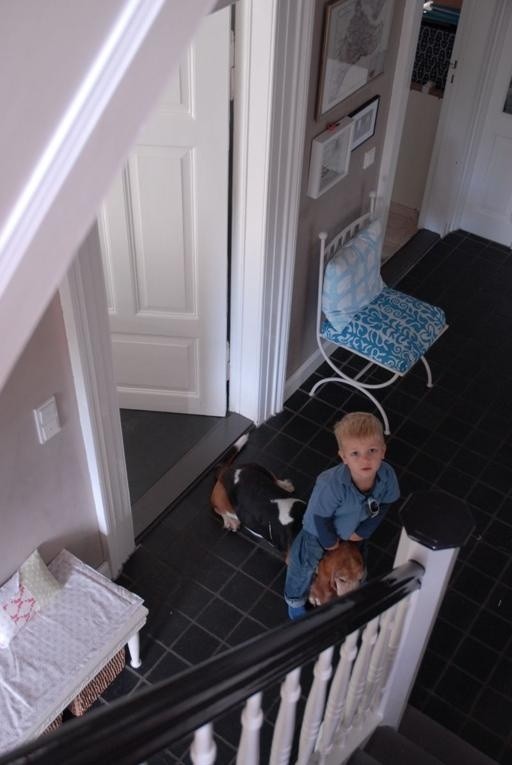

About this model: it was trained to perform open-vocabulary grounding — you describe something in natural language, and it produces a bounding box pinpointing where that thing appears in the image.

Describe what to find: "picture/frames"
[307,0,383,202]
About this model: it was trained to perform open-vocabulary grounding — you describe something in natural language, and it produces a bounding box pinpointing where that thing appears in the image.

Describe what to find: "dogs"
[208,431,369,609]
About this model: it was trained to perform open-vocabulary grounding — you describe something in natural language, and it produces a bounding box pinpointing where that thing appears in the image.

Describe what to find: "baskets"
[68,649,126,717]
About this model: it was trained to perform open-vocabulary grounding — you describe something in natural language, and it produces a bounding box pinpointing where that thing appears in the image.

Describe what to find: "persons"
[283,409,404,618]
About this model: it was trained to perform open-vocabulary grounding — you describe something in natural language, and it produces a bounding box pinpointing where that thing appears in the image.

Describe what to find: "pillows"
[1,550,63,645]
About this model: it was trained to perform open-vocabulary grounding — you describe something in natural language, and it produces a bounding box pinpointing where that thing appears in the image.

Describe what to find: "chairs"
[309,188,445,436]
